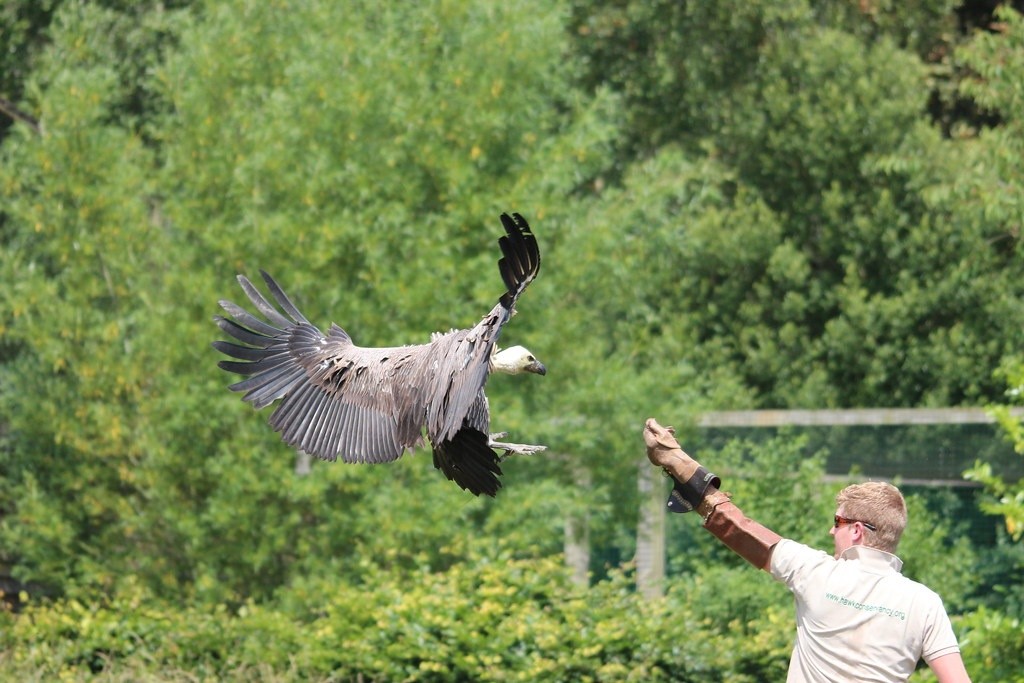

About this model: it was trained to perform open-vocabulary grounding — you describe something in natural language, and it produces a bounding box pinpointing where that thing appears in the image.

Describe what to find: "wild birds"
[215,209,546,497]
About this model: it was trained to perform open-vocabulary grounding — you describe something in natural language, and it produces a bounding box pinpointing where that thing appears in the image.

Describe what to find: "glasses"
[834,514,878,531]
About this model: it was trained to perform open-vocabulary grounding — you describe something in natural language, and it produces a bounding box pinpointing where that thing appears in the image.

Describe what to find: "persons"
[644,418,973,683]
